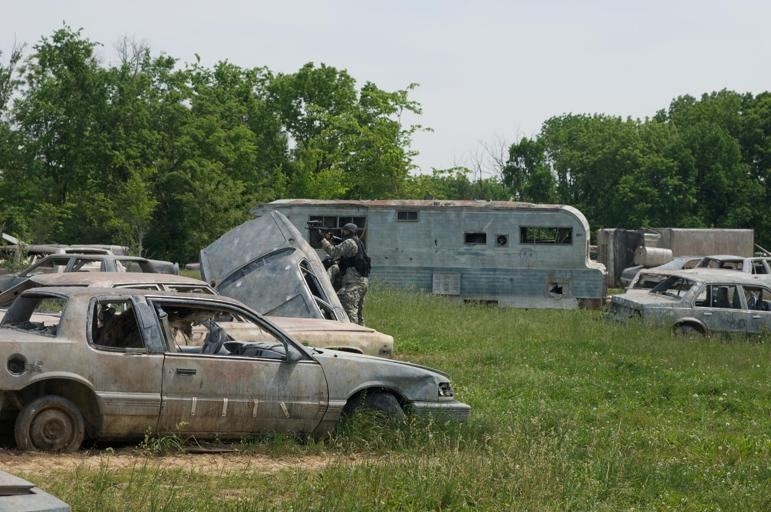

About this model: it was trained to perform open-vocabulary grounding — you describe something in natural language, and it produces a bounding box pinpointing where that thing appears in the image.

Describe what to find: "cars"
[604,254,771,342]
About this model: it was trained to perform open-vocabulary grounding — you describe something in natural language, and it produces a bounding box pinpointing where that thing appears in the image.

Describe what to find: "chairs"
[733,288,756,310]
[752,265,767,273]
[716,286,731,308]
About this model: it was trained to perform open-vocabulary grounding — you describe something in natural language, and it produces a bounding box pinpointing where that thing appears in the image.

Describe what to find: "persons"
[288,221,372,327]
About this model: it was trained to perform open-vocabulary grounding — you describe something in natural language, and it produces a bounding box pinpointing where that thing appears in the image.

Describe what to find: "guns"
[304,220,342,231]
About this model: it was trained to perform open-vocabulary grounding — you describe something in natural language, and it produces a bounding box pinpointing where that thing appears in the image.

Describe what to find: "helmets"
[342,223,359,233]
